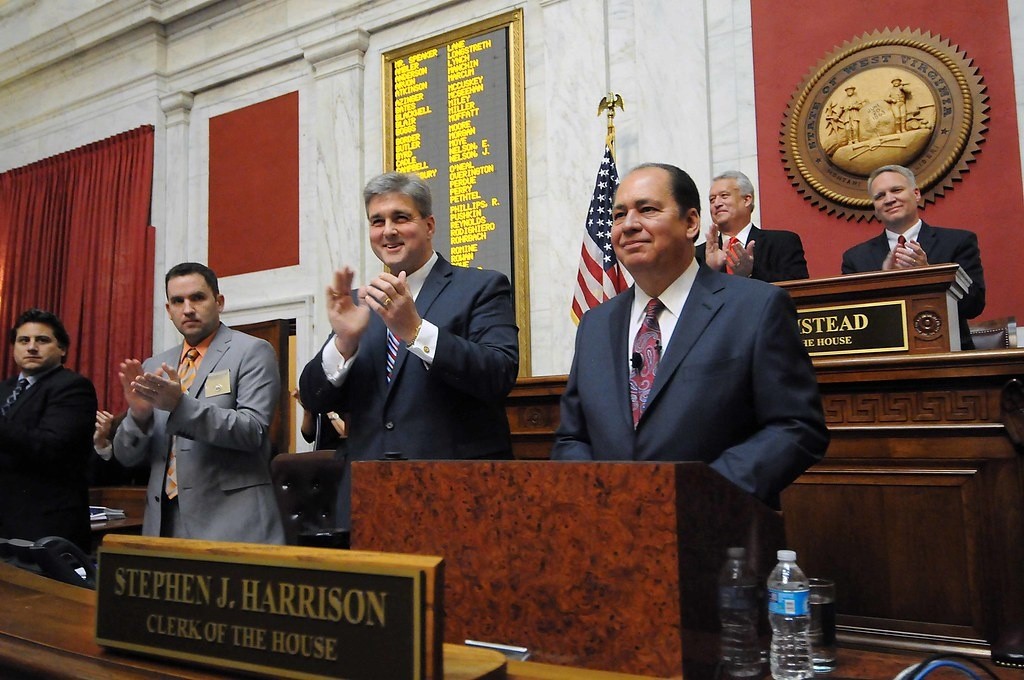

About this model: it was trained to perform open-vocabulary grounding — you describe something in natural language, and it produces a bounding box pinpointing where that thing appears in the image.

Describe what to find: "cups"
[809,578,836,673]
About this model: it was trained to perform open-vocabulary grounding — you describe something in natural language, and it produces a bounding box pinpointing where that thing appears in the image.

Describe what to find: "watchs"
[329,413,339,421]
[407,324,421,348]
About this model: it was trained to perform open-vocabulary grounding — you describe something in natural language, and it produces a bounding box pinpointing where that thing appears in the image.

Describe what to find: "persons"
[292,388,350,453]
[550,162,829,510]
[695,170,810,283]
[0,308,98,552]
[841,164,987,351]
[113,263,284,546]
[87,410,151,487]
[298,172,520,515]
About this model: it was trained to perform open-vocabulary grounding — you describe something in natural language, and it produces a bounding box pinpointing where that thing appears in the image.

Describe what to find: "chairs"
[271,450,340,544]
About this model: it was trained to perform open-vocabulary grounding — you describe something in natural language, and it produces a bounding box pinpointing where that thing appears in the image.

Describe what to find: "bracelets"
[748,274,752,278]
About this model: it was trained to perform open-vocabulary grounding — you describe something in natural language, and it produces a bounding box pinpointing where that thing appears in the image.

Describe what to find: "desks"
[0,559,660,680]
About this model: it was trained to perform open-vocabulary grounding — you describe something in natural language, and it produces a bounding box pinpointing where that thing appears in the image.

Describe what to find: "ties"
[385,331,400,382]
[0,378,29,417]
[165,349,200,500]
[726,237,738,275]
[630,298,666,429]
[898,236,906,250]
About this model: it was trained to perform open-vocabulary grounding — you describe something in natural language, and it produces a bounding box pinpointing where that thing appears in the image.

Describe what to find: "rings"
[736,263,740,266]
[919,249,922,255]
[383,298,390,305]
[333,291,340,296]
[912,260,916,264]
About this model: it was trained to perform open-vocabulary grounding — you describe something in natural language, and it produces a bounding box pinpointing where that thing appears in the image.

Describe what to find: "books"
[89,506,126,521]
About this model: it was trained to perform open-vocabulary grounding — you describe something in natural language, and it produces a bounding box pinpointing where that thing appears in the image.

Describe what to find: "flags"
[570,139,628,326]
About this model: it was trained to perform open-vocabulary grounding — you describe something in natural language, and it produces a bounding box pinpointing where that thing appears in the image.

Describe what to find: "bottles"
[764,550,813,680]
[720,547,761,676]
